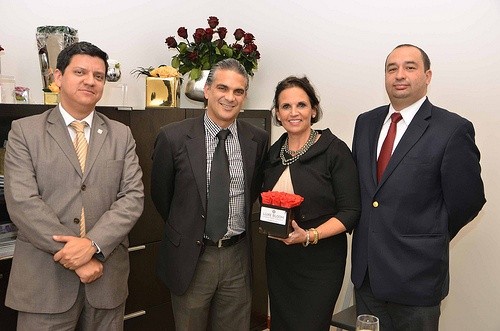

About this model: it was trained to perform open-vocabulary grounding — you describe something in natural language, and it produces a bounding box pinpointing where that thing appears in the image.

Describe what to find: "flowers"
[134,62,181,110]
[261,190,305,208]
[166,18,261,110]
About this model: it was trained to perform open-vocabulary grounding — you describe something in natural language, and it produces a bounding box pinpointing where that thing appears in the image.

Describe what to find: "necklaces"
[280,128,318,166]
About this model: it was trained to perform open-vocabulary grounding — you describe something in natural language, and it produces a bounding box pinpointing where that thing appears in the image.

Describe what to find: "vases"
[146,76,180,110]
[259,204,299,239]
[183,70,224,109]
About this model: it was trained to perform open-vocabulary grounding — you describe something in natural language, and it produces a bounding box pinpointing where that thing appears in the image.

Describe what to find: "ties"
[68,120,88,237]
[377,112,403,185]
[204,130,229,243]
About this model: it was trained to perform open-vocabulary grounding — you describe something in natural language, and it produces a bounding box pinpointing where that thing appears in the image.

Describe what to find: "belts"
[204,232,246,247]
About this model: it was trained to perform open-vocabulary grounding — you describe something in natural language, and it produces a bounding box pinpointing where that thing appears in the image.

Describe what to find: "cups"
[356,314,379,331]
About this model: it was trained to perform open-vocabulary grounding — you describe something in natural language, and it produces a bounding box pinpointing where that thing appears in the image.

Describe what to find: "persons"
[352,44,487,331]
[258,75,362,331]
[149,58,272,331]
[3,42,144,331]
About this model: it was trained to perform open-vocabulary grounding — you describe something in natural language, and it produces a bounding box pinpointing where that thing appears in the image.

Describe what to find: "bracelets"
[309,228,319,244]
[91,241,101,253]
[303,229,310,247]
